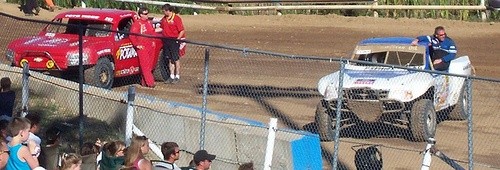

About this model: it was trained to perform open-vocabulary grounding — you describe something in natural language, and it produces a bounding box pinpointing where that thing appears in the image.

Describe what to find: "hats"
[194,150,216,163]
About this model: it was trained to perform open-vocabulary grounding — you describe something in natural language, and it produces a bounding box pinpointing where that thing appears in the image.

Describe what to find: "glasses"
[437,33,446,36]
[204,159,211,162]
[142,13,148,15]
[2,150,11,156]
[175,151,179,154]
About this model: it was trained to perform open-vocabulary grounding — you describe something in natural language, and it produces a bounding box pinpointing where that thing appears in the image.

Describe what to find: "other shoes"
[36,7,41,13]
[175,75,180,80]
[170,74,175,80]
[51,6,55,11]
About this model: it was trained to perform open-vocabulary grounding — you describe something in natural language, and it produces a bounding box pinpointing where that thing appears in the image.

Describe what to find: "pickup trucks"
[4,8,170,90]
[314,37,475,141]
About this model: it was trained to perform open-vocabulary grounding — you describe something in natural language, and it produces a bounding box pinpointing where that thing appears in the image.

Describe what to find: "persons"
[57,152,83,170]
[80,143,98,170]
[0,136,11,170]
[0,76,17,121]
[22,112,42,158]
[129,7,158,89]
[410,26,457,72]
[4,115,39,170]
[37,126,66,170]
[160,141,182,170]
[192,150,212,170]
[93,137,128,170]
[155,3,185,84]
[0,119,10,143]
[123,135,154,170]
[24,0,41,16]
